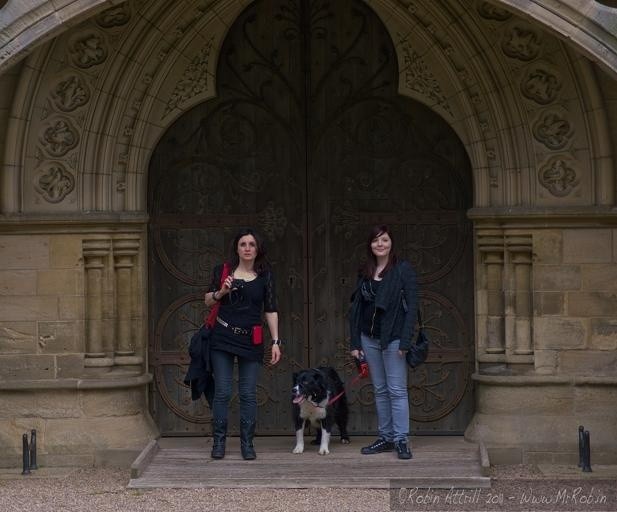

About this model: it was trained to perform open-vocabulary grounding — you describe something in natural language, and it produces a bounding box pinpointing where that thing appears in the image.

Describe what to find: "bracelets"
[271,339,281,345]
[212,291,220,301]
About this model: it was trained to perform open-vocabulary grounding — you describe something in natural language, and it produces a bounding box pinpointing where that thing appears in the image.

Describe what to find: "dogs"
[291,367,350,455]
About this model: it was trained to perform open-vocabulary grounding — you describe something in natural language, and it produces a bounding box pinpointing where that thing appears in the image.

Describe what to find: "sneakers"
[396,440,412,459]
[361,440,395,454]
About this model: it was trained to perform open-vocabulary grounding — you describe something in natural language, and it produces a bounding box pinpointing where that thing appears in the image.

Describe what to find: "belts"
[217,316,250,336]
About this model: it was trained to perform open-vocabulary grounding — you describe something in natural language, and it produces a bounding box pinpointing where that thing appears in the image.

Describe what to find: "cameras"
[230,279,244,289]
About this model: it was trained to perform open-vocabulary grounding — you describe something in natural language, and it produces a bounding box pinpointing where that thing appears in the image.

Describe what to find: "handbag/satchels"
[406,333,428,368]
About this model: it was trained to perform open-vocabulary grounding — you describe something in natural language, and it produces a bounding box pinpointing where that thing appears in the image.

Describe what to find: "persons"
[205,229,282,460]
[350,224,418,459]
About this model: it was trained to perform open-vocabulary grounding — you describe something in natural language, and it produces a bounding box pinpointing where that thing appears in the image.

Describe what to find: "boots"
[211,418,227,458]
[240,419,256,460]
